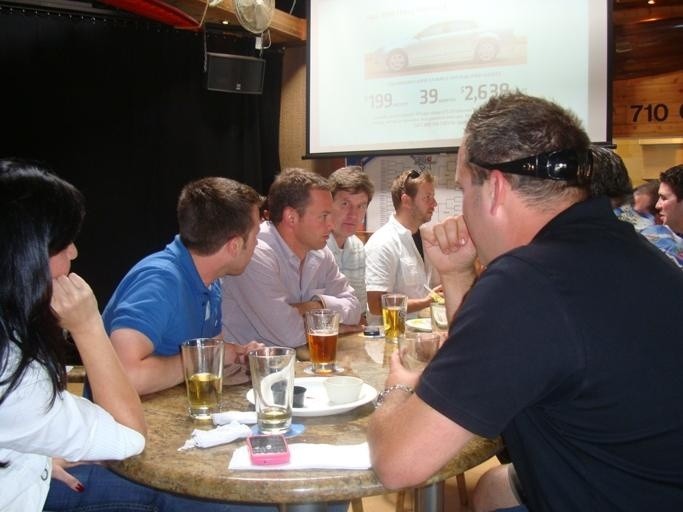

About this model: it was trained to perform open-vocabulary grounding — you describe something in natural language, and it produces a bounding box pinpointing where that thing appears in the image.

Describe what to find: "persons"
[223,167,364,348]
[326,166,373,326]
[365,93,683,512]
[365,170,443,325]
[83,176,267,403]
[593,147,683,268]
[0,159,278,512]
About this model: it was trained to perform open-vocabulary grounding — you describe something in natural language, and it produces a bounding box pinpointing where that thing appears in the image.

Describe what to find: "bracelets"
[374,384,414,409]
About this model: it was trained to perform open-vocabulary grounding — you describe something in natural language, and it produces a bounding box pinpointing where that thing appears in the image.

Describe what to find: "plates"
[246,375,378,418]
[405,318,432,332]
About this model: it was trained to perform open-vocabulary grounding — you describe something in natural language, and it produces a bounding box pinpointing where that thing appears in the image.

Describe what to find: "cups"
[398,331,440,372]
[363,299,381,335]
[248,346,297,436]
[381,293,408,345]
[305,308,340,375]
[181,337,225,420]
[293,386,306,408]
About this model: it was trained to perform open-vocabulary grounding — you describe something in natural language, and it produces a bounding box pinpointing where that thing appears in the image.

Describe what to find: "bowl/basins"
[322,376,363,404]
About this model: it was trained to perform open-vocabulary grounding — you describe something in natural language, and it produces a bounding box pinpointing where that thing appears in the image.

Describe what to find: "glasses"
[402,170,420,185]
[469,148,593,180]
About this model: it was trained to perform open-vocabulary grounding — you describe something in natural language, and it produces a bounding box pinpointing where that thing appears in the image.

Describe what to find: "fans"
[232,0,277,34]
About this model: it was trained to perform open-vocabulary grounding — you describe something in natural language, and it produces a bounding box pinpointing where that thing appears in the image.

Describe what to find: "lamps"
[206,52,265,95]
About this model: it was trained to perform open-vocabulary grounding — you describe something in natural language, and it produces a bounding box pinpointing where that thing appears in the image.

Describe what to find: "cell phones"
[247,433,291,465]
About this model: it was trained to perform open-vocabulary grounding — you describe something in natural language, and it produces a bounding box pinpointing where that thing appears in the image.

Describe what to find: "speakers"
[203,52,267,95]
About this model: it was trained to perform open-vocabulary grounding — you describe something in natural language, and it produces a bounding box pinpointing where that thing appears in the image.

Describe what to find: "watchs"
[83,176,267,403]
